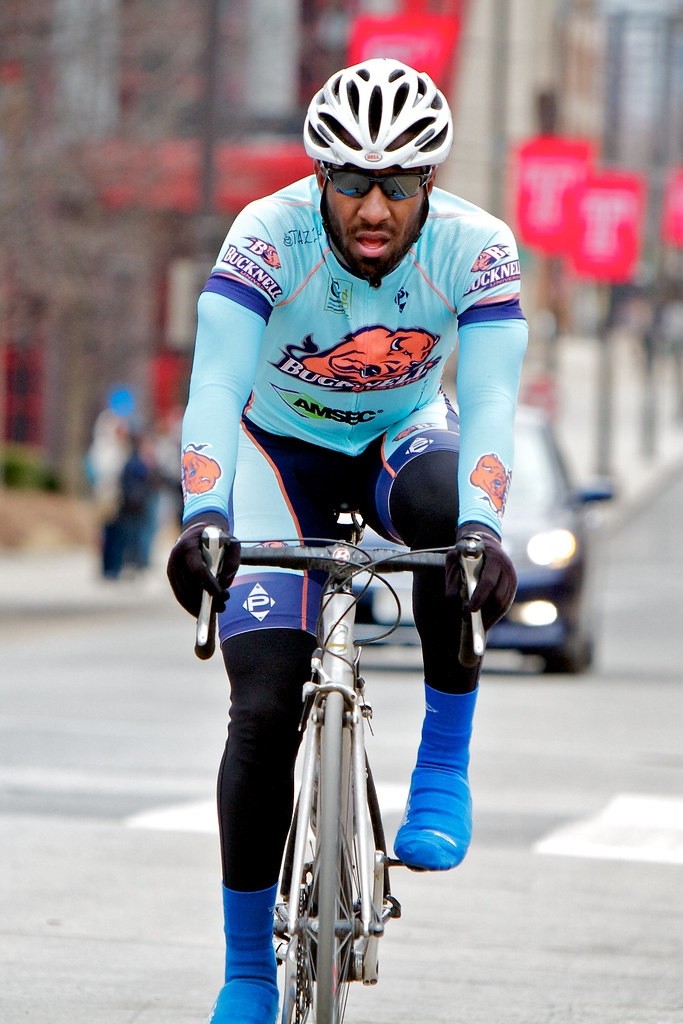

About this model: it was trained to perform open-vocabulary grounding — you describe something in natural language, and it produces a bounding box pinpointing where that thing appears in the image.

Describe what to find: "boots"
[393,682,480,871]
[208,881,279,1024]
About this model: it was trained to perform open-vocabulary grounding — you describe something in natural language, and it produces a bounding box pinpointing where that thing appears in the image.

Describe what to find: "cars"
[351,405,616,676]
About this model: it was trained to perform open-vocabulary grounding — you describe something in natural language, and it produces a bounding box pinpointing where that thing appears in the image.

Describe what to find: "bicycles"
[194,524,491,1024]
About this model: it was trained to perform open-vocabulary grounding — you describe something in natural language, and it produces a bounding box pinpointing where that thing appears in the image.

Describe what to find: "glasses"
[319,160,437,201]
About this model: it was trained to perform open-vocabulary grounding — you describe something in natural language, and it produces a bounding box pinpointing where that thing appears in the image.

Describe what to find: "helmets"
[302,57,454,171]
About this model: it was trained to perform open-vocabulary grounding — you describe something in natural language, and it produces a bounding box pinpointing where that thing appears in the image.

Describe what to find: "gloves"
[167,512,241,619]
[445,522,519,630]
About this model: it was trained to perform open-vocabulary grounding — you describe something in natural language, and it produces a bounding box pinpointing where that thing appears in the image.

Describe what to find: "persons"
[165,57,529,1024]
[86,391,178,581]
